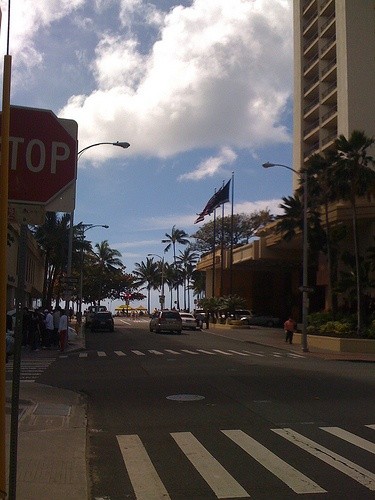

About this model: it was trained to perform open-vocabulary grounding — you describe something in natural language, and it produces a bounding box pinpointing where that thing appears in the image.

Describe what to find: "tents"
[115,305,147,317]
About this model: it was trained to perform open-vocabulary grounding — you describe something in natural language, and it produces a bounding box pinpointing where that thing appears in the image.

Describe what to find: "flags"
[194,178,232,223]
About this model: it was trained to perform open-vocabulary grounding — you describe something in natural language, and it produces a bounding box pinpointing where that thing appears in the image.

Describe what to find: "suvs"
[221,309,257,324]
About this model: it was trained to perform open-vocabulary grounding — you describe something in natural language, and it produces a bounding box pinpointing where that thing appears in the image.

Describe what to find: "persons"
[21,306,73,353]
[193,309,197,319]
[154,308,156,314]
[136,309,147,320]
[285,317,295,344]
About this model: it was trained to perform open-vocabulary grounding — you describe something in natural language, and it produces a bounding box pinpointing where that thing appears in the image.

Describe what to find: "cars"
[194,308,212,320]
[178,312,198,330]
[150,309,183,335]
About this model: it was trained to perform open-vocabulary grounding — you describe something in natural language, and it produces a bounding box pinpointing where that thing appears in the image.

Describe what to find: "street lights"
[77,224,110,324]
[261,161,309,352]
[64,140,132,318]
[147,253,165,309]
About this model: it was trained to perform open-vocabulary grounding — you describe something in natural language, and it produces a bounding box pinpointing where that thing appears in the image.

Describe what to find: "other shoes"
[34,348,40,351]
[57,350,64,353]
[43,347,49,349]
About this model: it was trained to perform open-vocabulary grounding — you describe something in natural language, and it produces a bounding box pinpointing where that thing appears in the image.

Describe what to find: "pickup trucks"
[85,304,113,325]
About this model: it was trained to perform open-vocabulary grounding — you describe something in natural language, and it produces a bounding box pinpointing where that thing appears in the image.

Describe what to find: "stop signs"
[0,103,78,206]
